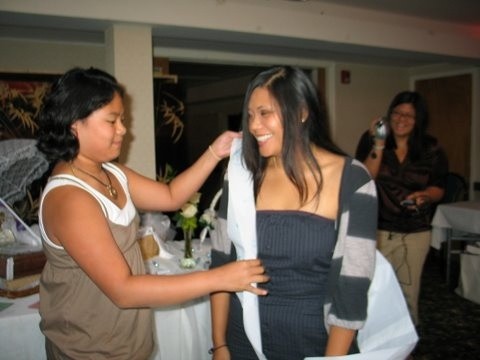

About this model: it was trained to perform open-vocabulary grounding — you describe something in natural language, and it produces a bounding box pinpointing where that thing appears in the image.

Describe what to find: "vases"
[183,226,194,259]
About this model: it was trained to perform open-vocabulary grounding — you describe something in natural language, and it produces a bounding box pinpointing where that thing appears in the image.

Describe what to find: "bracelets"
[209,145,221,160]
[209,343,228,354]
[374,146,384,150]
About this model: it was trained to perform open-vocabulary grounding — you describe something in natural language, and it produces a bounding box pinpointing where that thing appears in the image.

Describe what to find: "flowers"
[172,190,201,231]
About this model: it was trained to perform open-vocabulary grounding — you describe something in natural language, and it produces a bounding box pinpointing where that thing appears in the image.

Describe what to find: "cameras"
[375,121,386,137]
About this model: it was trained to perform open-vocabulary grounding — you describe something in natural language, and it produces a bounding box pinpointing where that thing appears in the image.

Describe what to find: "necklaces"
[70,164,118,199]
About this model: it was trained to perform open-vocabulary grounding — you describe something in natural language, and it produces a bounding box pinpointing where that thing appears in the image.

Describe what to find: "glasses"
[390,110,416,121]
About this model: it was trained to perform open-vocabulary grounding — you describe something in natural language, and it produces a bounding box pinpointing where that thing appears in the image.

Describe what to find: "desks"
[430,201,480,291]
[0,235,419,360]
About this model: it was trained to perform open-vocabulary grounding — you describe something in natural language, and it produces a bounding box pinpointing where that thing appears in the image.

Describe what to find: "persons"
[355,91,446,327]
[208,65,378,360]
[34,66,270,360]
